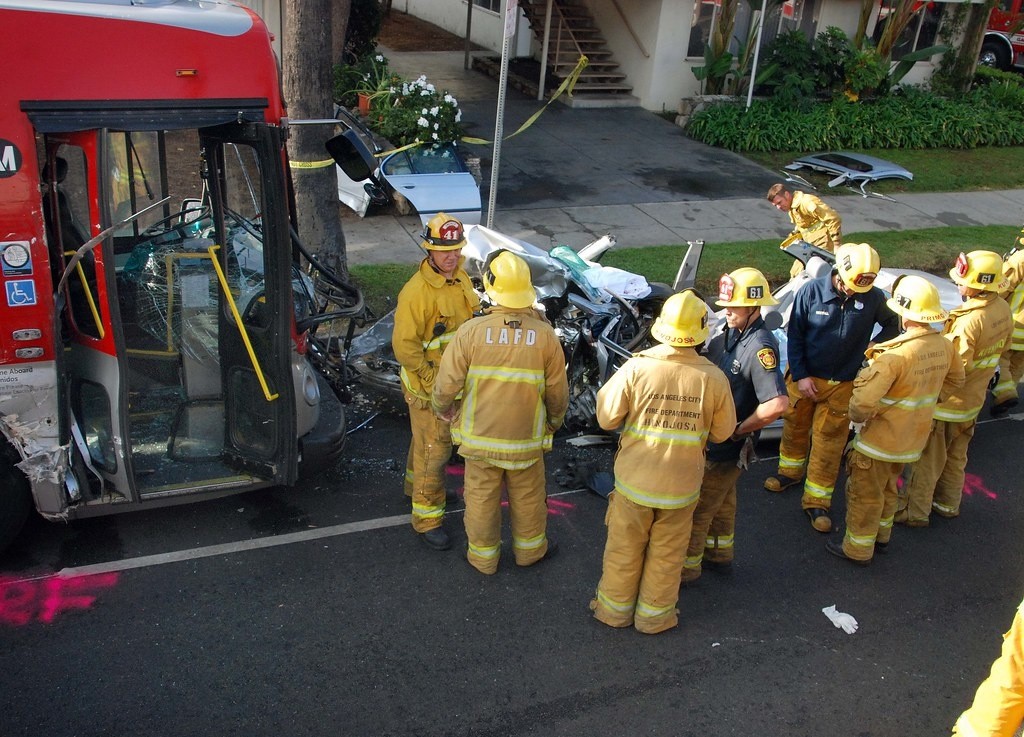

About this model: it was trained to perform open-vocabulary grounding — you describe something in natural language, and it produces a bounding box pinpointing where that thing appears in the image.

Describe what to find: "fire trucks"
[692,0,1024,72]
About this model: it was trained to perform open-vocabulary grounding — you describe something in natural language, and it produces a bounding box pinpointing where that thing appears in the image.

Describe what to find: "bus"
[0,1,388,565]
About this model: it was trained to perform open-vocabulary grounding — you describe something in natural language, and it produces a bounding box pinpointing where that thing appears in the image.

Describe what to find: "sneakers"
[877,541,888,549]
[826,542,871,565]
[764,474,800,491]
[419,528,452,550]
[806,508,831,532]
[407,485,461,503]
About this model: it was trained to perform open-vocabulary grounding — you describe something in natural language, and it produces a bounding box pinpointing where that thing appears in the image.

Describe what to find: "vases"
[358,92,377,116]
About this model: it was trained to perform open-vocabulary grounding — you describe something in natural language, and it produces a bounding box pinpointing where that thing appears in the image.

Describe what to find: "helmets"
[949,250,1009,292]
[836,242,881,293]
[715,267,780,307]
[483,252,536,309]
[886,275,950,323]
[651,287,709,347]
[421,212,467,251]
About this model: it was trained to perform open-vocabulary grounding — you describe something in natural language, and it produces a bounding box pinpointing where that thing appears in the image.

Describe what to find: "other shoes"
[894,511,928,527]
[702,559,732,569]
[991,398,1018,415]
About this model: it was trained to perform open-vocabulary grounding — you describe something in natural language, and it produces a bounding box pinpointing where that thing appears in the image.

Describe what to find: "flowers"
[363,51,465,159]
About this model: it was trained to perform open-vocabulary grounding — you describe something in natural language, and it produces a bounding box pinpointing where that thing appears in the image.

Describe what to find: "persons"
[431,249,570,575]
[950,598,1024,736]
[679,267,789,583]
[825,274,966,566]
[767,182,842,278]
[393,210,482,551]
[989,224,1024,415]
[589,289,737,635]
[891,249,1014,528]
[764,241,902,532]
